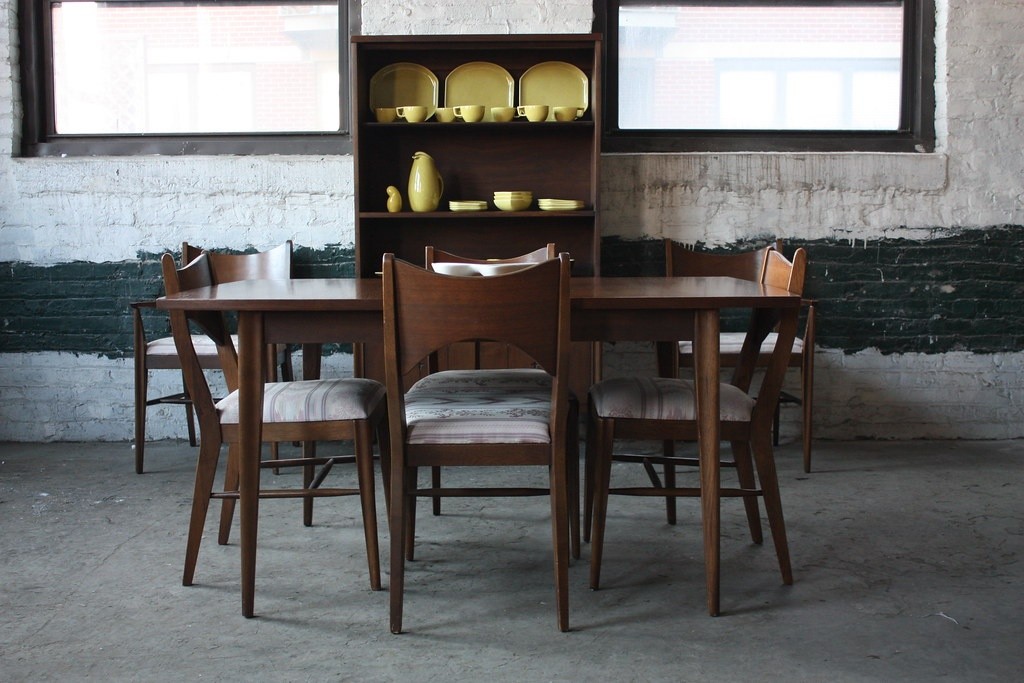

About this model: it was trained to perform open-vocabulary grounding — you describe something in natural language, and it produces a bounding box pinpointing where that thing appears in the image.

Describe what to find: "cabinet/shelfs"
[355,342,597,422]
[349,32,604,276]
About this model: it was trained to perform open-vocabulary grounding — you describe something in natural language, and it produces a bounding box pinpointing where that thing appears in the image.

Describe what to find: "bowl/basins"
[493,192,533,210]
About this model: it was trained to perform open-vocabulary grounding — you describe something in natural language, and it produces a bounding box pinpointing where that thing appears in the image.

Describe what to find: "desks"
[156,277,802,617]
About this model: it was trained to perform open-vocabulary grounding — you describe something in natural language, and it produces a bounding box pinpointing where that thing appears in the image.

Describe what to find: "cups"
[436,108,455,122]
[373,106,396,121]
[492,107,519,121]
[453,105,484,122]
[394,106,427,122]
[553,107,584,120]
[517,106,548,121]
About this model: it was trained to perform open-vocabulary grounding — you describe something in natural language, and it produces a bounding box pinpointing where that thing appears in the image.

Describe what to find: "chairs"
[584,246,807,592]
[665,237,820,474]
[425,242,567,514]
[160,248,393,592]
[380,250,580,634]
[130,240,301,476]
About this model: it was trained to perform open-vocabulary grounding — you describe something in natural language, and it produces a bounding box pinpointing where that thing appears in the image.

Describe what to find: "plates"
[369,63,438,120]
[520,61,588,121]
[449,201,487,209]
[538,199,584,211]
[445,62,514,121]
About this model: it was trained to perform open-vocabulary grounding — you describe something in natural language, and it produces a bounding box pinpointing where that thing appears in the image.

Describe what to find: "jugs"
[409,152,443,212]
